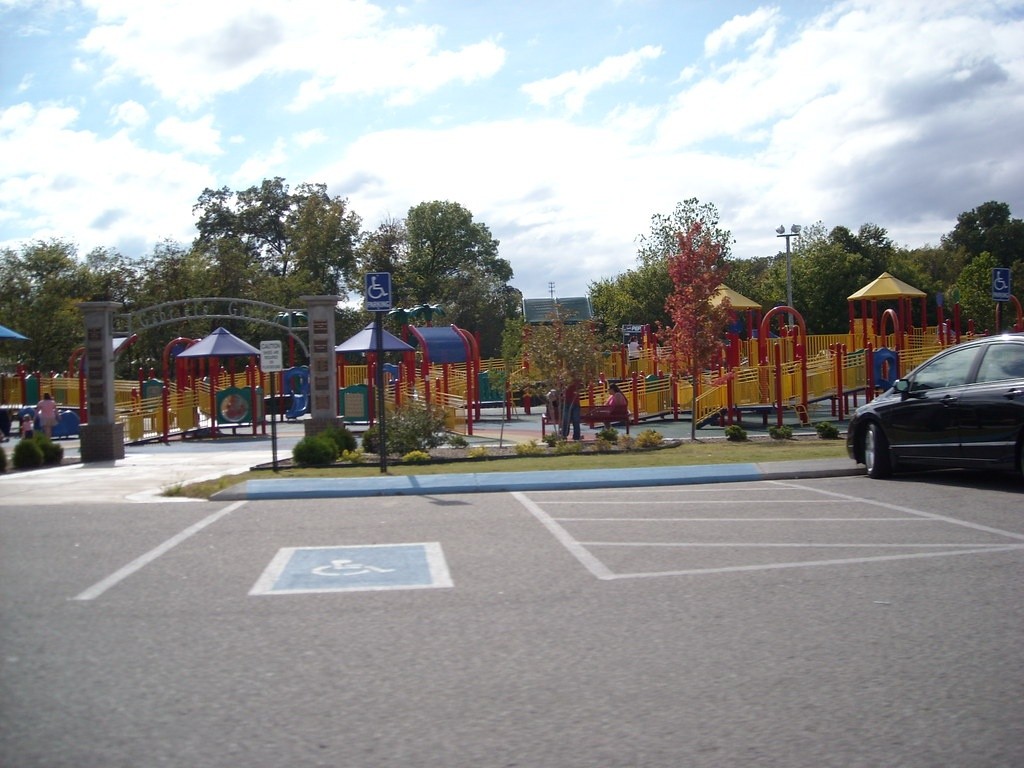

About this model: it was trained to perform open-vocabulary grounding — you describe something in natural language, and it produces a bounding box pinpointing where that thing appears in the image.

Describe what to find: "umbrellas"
[0,325,33,342]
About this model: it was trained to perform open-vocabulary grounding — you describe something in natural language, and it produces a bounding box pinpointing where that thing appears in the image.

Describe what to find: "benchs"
[541,404,632,442]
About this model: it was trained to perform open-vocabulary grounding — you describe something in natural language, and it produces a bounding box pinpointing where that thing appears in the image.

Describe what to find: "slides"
[879,377,897,390]
[286,392,308,419]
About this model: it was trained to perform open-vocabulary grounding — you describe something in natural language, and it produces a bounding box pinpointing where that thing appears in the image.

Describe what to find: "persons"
[35,392,61,438]
[21,414,34,439]
[628,336,641,378]
[561,368,585,443]
[602,383,629,433]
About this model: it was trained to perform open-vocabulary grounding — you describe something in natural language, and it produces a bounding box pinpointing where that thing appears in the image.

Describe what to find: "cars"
[846,331,1024,479]
